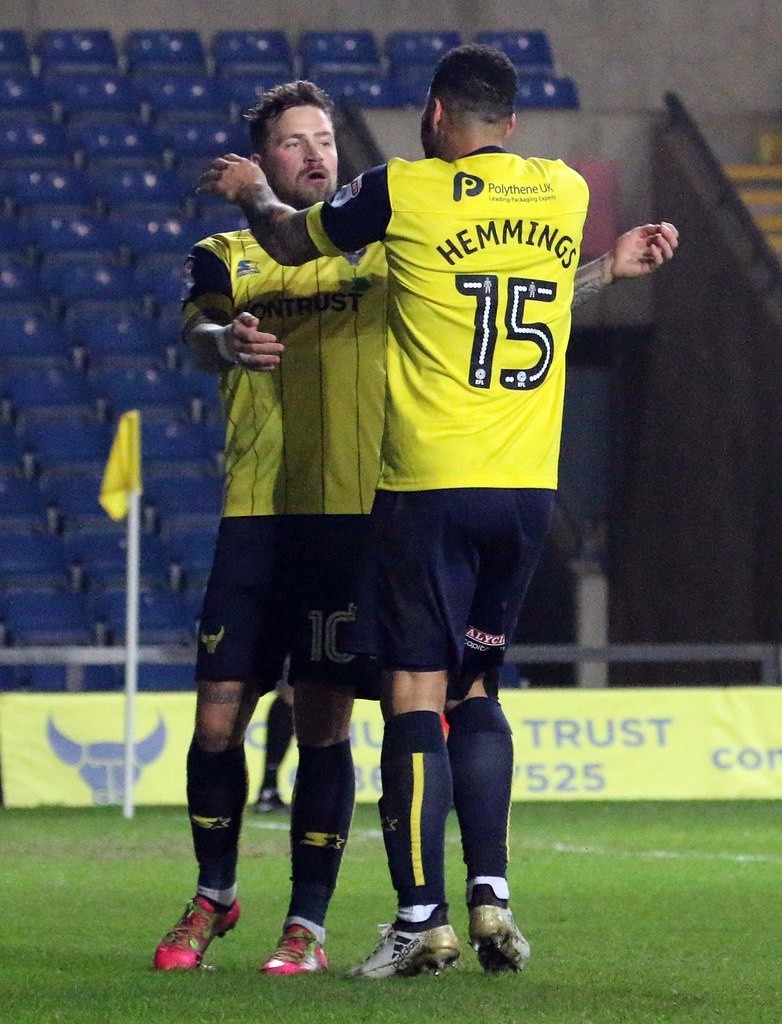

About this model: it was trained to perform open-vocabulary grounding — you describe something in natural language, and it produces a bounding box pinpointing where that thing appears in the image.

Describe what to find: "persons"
[253,655,294,815]
[149,78,678,975]
[195,41,591,979]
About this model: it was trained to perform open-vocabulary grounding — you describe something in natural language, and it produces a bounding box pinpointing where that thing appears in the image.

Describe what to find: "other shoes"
[254,783,284,813]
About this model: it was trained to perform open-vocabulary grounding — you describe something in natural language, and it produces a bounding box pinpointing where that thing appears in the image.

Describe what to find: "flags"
[97,410,142,523]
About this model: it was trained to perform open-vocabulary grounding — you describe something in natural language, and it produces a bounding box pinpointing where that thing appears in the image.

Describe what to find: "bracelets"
[215,327,234,361]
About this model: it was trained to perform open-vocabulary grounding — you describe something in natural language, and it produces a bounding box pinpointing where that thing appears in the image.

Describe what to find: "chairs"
[0,30,590,694]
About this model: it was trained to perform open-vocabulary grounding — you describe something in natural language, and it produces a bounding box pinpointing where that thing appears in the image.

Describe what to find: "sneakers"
[257,923,328,976]
[466,884,530,973]
[344,902,461,982]
[154,895,241,971]
